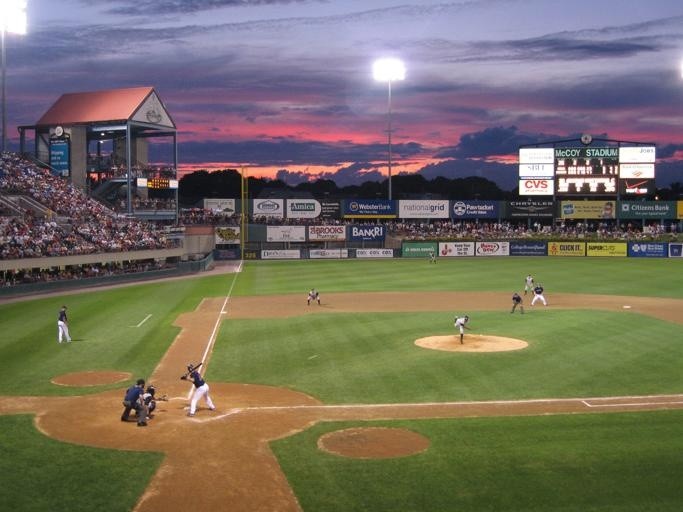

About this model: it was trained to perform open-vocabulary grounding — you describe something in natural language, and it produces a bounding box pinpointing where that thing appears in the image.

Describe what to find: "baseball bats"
[182,363,203,377]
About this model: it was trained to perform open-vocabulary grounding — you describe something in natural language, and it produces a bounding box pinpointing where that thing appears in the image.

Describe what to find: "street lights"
[0,0,29,151]
[372,56,406,200]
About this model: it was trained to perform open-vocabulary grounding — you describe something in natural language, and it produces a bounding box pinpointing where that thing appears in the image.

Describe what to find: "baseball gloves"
[159,394,170,401]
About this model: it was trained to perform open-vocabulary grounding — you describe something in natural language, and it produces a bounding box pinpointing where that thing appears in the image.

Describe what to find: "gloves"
[181,376,187,379]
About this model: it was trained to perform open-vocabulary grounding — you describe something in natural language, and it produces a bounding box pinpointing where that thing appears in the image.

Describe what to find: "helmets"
[136,379,145,388]
[146,386,156,396]
[188,364,194,372]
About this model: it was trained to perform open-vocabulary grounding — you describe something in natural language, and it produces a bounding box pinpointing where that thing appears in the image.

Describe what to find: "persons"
[56,306,71,343]
[510,292,523,314]
[135,385,169,419]
[306,288,320,305]
[352,220,682,241]
[248,213,351,226]
[180,364,215,416]
[429,253,436,264]
[602,202,613,219]
[523,273,535,296]
[178,207,244,226]
[530,283,547,306]
[0,148,177,288]
[119,378,147,426]
[454,315,468,344]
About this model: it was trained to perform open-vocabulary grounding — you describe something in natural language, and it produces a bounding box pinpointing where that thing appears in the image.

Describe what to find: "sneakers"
[187,412,195,416]
[137,422,147,426]
[122,418,128,421]
